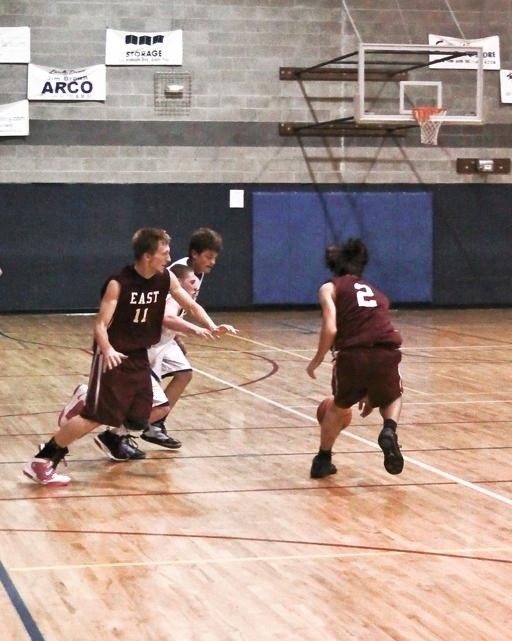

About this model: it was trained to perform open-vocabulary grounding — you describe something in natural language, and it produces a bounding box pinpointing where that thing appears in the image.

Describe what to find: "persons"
[140,227,222,448]
[306,239,404,478]
[39,264,221,471]
[22,227,239,487]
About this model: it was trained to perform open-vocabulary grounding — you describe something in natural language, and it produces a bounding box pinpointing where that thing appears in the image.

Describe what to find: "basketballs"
[317,399,353,429]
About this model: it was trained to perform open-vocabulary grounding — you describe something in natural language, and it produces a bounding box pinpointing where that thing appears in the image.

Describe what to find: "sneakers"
[140,422,182,450]
[57,382,90,429]
[21,443,72,488]
[377,426,404,477]
[309,455,338,479]
[120,435,146,461]
[93,428,128,461]
